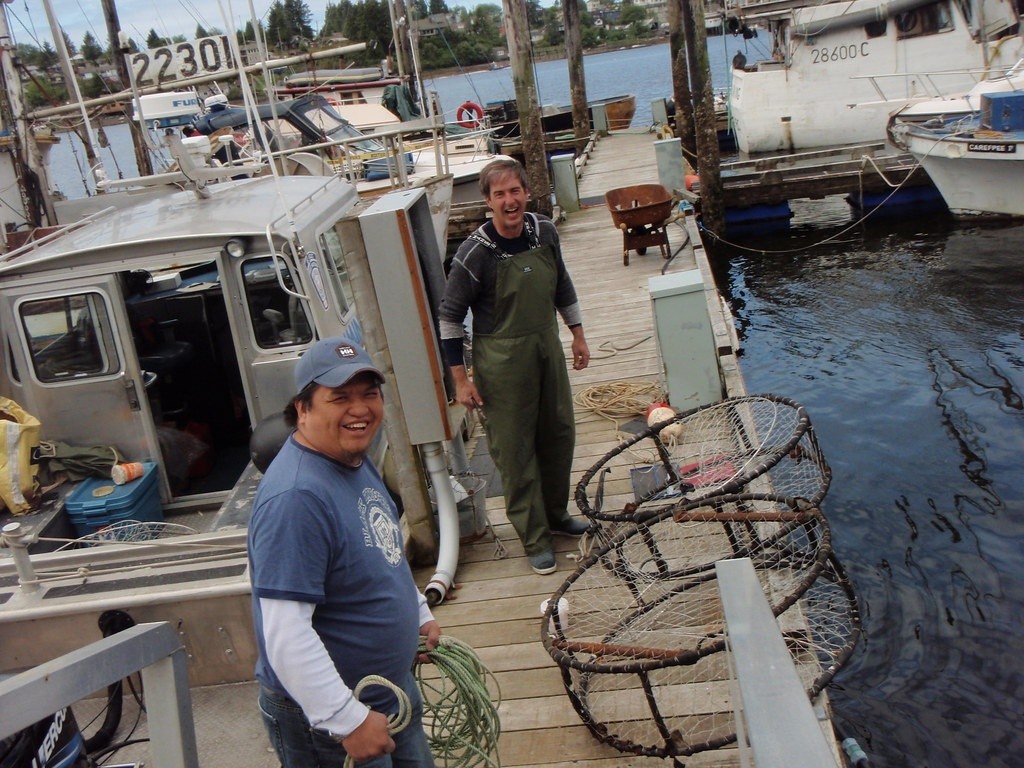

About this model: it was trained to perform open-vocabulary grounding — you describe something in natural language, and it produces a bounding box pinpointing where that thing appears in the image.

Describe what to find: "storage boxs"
[131,91,202,130]
[63,461,167,548]
[364,153,415,182]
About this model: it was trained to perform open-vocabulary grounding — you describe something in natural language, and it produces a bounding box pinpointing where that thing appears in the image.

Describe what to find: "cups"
[111,461,144,485]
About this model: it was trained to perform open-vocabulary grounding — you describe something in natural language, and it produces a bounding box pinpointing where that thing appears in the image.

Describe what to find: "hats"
[293,337,386,394]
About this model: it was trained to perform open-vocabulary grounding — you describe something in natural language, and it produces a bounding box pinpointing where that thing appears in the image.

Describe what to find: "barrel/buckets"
[159,421,212,477]
[426,476,487,545]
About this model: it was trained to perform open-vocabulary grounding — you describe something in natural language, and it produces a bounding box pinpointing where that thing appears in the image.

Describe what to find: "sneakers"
[528,549,557,575]
[549,517,590,538]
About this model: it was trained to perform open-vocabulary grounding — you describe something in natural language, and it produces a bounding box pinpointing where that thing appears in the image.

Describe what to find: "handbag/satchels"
[32,439,129,487]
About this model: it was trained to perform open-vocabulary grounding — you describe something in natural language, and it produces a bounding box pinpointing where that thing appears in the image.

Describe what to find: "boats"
[887,68,1024,225]
[481,91,635,140]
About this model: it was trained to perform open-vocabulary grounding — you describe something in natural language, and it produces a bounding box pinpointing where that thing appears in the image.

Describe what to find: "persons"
[438,160,602,575]
[247,337,442,768]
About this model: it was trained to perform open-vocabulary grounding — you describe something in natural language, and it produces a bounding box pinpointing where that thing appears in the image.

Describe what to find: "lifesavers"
[457,102,484,128]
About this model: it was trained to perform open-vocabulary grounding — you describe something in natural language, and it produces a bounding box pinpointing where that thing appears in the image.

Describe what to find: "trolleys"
[603,182,682,267]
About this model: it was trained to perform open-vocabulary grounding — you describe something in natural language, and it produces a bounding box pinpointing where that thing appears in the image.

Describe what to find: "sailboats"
[1,1,528,244]
